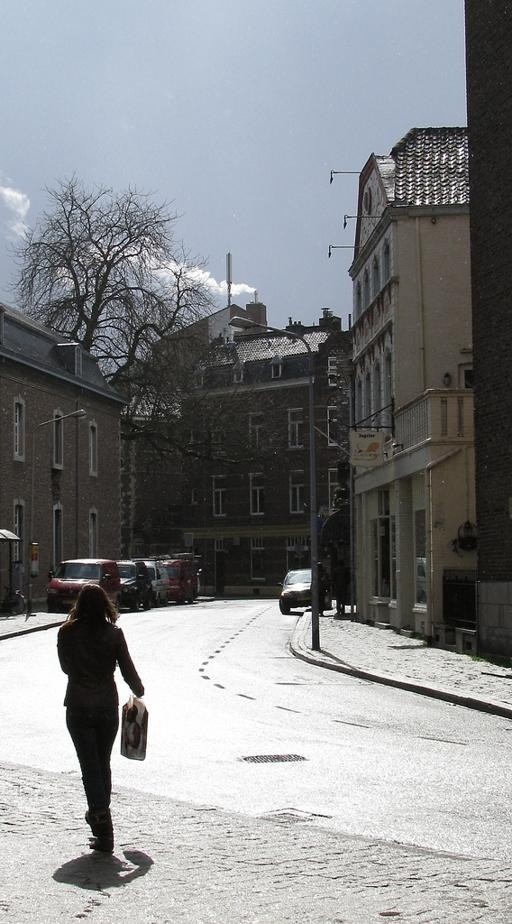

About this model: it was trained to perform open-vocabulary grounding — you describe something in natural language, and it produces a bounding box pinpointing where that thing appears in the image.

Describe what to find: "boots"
[90,809,117,853]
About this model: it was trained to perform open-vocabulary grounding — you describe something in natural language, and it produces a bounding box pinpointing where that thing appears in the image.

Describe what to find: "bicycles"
[0,585,25,615]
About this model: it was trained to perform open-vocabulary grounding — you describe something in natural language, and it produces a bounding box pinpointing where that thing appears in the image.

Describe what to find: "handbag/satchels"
[120,700,150,762]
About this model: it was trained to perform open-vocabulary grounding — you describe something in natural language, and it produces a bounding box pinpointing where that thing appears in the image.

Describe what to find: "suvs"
[45,550,205,613]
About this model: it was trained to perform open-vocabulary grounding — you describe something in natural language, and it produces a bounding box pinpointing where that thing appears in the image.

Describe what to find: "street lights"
[225,313,324,651]
[22,407,87,614]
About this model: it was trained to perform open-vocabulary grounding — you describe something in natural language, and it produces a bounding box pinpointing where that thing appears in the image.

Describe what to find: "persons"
[309,561,331,618]
[334,558,348,617]
[58,583,145,854]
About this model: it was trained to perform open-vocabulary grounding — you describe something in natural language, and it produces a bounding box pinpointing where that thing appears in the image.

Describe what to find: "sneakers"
[84,809,97,839]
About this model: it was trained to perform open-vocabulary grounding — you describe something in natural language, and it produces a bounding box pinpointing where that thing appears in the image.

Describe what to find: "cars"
[275,566,334,615]
